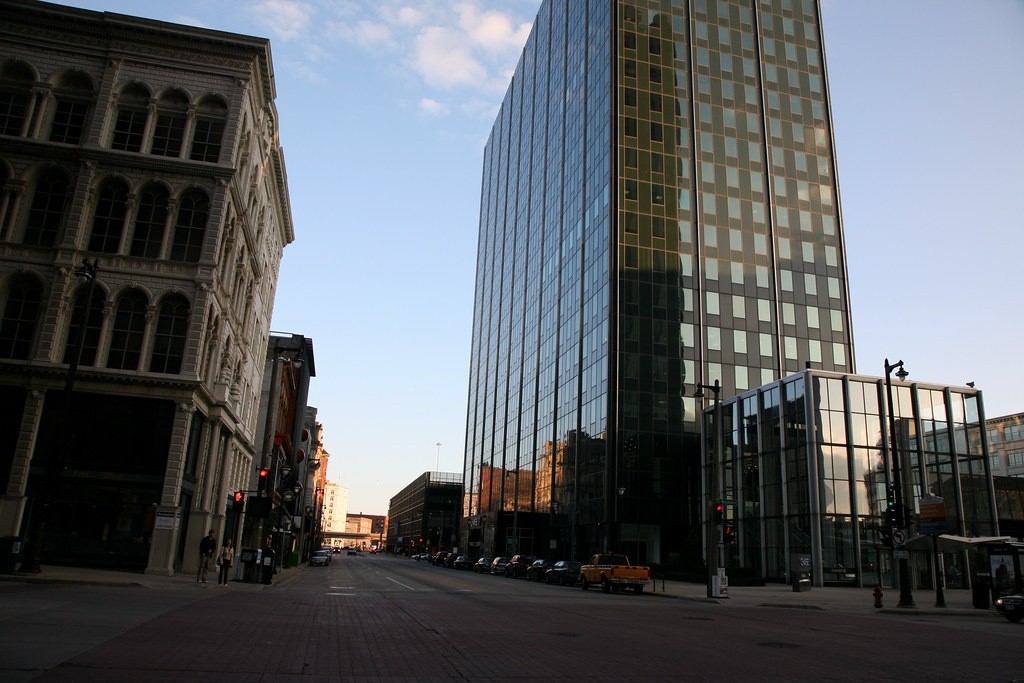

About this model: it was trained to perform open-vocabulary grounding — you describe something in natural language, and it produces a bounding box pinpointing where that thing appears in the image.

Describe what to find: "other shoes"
[224,584,227,586]
[197,580,201,583]
[219,583,224,586]
[203,579,208,582]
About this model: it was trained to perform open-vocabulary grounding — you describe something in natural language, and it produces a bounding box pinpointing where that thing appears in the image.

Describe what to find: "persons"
[196,529,216,583]
[218,538,234,585]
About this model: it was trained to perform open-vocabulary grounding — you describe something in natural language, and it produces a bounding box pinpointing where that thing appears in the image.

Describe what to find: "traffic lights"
[234,492,244,502]
[905,504,914,527]
[887,489,894,508]
[725,524,734,534]
[258,469,266,489]
[716,504,722,523]
[886,506,898,524]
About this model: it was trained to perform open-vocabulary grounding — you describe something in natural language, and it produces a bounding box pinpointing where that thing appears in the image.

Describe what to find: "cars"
[369,548,376,553]
[312,546,340,565]
[349,548,356,554]
[412,551,473,569]
[995,584,1024,622]
[473,555,581,586]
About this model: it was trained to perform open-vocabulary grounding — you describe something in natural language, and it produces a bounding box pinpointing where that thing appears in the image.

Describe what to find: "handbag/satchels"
[217,556,220,565]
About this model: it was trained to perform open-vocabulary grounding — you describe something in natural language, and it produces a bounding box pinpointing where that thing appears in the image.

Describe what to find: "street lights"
[273,464,301,574]
[885,358,916,607]
[252,346,304,582]
[615,487,626,553]
[506,469,519,556]
[693,378,720,598]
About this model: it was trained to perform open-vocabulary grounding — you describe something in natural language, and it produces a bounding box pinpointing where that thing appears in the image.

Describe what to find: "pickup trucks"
[578,553,650,594]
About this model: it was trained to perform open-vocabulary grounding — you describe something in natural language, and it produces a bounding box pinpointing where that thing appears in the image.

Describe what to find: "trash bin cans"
[258,549,276,584]
[0,536,24,575]
[292,551,300,566]
[792,580,810,592]
